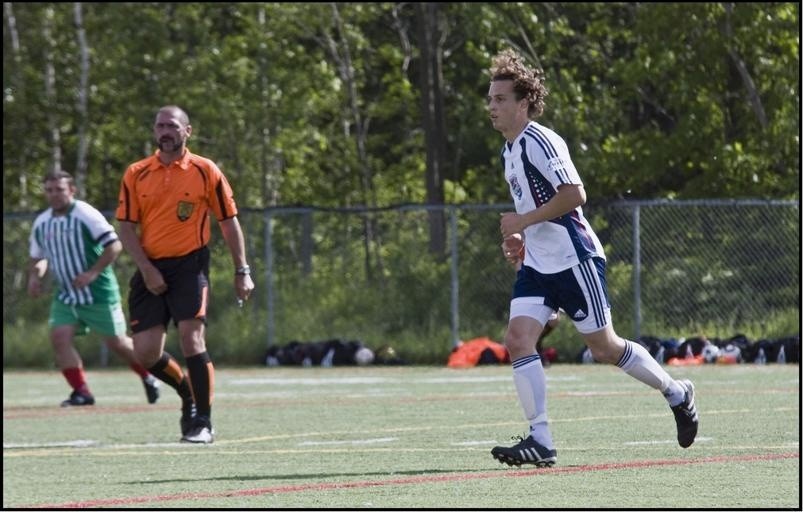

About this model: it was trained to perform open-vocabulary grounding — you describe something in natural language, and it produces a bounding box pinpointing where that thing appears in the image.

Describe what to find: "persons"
[116,104,255,444]
[488,48,699,469]
[27,171,160,407]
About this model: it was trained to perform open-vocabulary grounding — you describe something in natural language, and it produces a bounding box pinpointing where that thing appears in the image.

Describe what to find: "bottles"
[776,346,787,364]
[757,346,766,366]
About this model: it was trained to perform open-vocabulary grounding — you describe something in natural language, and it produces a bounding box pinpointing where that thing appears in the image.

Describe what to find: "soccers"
[702,346,720,363]
[356,349,374,368]
[377,345,396,364]
[721,345,741,363]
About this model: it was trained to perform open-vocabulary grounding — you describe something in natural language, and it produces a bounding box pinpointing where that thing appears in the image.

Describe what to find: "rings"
[506,252,511,256]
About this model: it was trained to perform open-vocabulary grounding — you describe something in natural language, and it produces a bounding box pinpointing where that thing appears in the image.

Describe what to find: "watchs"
[235,265,250,275]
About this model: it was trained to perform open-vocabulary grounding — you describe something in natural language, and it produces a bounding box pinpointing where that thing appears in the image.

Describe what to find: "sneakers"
[181,399,214,444]
[670,378,699,448]
[490,436,557,467]
[143,375,161,405]
[60,389,94,405]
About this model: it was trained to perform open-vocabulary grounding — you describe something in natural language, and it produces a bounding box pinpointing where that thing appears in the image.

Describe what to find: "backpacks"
[266,339,402,367]
[577,334,800,363]
